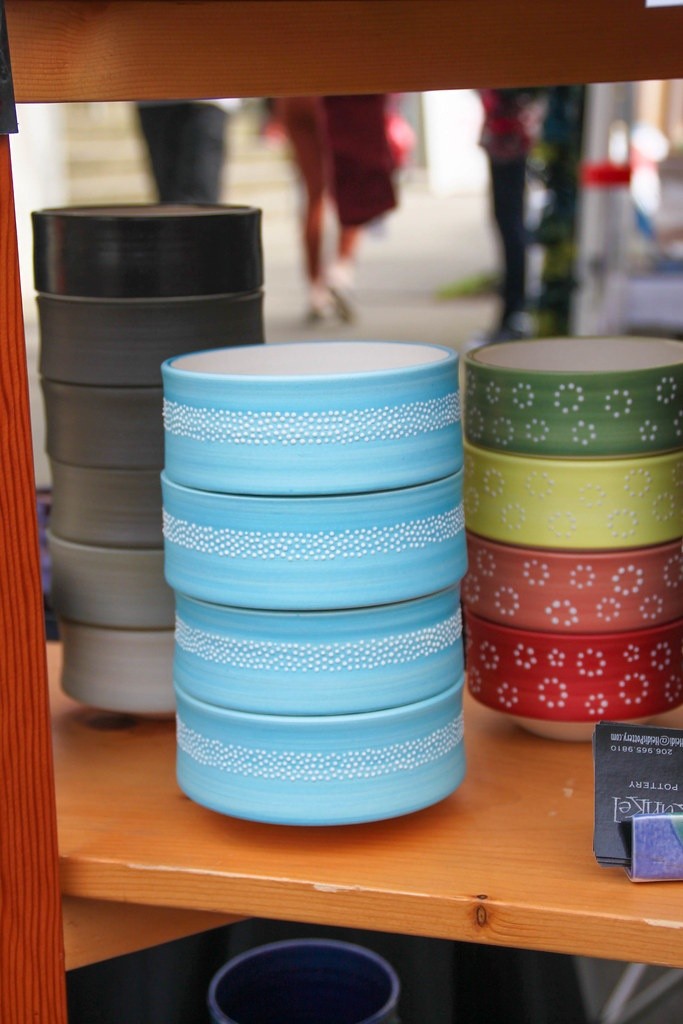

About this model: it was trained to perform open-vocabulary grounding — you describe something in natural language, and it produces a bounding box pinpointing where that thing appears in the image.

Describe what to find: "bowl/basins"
[461,530,683,634]
[45,461,165,551]
[57,613,176,718]
[34,291,265,388]
[464,335,683,457]
[463,440,682,551]
[207,943,399,1024]
[157,471,468,610]
[45,529,176,635]
[160,338,465,496]
[174,681,466,828]
[31,204,266,297]
[462,604,680,741]
[171,589,465,717]
[38,377,166,470]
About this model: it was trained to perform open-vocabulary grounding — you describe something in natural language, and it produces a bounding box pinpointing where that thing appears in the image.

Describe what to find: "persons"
[475,83,589,344]
[262,96,417,327]
[133,98,245,202]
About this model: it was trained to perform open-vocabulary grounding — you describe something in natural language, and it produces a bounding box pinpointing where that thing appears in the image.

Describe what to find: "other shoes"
[300,283,363,329]
